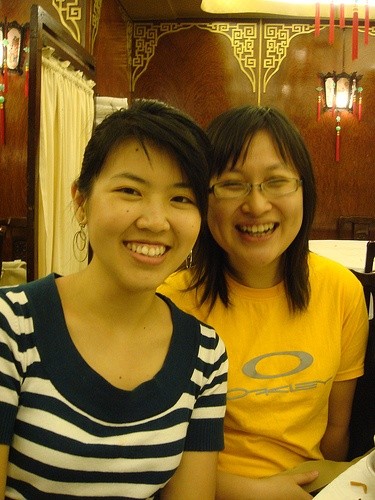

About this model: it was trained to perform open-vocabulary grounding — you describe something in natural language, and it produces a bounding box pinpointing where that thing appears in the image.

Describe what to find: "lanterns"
[0,17,32,146]
[315,69,364,162]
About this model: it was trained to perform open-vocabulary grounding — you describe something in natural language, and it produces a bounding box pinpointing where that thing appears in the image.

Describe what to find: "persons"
[0,98,229,500]
[157,104,370,500]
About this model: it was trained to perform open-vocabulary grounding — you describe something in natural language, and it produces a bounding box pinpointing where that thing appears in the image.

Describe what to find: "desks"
[308,240,375,333]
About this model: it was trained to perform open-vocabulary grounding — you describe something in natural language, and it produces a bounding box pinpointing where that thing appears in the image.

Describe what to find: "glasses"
[210,177,303,199]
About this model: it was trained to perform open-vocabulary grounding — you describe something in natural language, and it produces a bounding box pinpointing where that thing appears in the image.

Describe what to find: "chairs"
[0,217,27,261]
[347,268,375,462]
[336,216,375,240]
